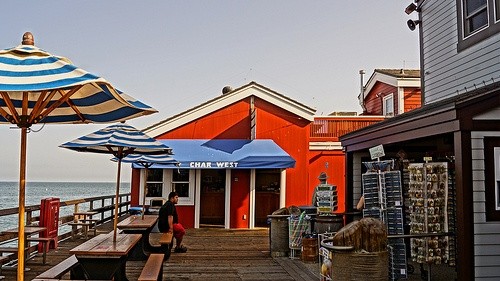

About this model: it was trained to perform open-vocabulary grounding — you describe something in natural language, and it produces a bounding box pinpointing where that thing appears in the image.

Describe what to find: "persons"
[312,172,328,205]
[357,195,364,210]
[158,192,187,253]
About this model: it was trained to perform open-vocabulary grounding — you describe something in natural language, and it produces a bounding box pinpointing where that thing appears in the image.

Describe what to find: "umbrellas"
[0,30,158,281]
[58,121,181,242]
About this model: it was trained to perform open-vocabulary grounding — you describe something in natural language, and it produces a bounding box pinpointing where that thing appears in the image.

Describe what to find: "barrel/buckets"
[301,233,319,264]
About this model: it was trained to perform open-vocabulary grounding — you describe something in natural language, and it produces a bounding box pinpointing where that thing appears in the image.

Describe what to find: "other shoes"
[174,245,188,253]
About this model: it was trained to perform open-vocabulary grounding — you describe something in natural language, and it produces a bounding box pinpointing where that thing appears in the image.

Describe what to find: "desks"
[74,211,97,239]
[117,215,159,261]
[0,227,46,271]
[70,233,142,281]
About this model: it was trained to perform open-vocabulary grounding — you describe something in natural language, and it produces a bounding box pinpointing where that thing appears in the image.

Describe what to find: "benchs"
[0,248,28,253]
[108,228,123,234]
[79,219,98,222]
[138,254,164,281]
[35,254,78,278]
[159,233,173,243]
[26,238,51,241]
[68,223,90,226]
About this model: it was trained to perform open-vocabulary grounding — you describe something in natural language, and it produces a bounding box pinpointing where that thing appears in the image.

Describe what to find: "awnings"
[132,139,295,168]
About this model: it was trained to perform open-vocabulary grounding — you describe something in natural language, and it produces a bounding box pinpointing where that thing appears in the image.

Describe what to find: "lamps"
[404,3,417,15]
